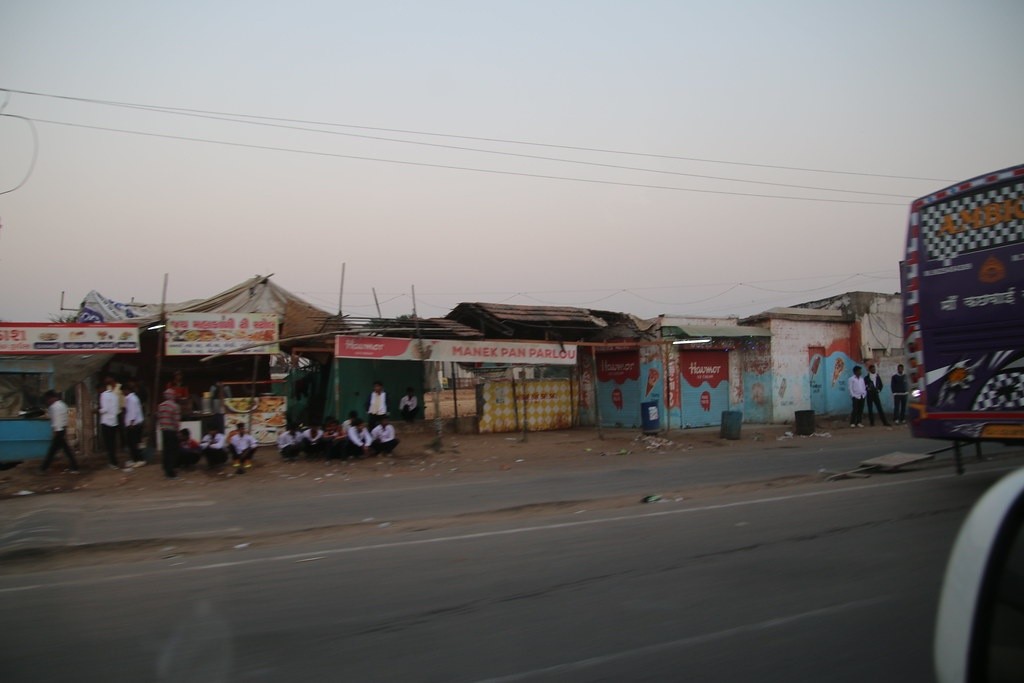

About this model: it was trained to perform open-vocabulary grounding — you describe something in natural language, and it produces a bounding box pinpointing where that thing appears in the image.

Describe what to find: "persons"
[157,388,180,455]
[848,366,867,428]
[363,381,391,432]
[864,365,891,426]
[891,364,909,425]
[93,375,147,472]
[277,411,400,462]
[176,423,258,472]
[38,388,79,475]
[399,385,419,424]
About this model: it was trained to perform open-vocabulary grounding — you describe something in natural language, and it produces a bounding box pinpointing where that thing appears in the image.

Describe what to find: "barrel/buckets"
[640,401,660,430]
[794,409,816,435]
[720,410,743,440]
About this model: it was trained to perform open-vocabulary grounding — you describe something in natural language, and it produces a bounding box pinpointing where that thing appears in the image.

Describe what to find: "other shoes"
[132,461,146,467]
[871,422,874,426]
[857,423,864,427]
[883,422,892,426]
[850,424,855,428]
[244,461,252,467]
[233,458,239,467]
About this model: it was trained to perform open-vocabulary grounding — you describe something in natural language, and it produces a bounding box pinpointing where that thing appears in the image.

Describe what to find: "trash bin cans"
[720,410,743,440]
[795,409,815,435]
[640,399,660,430]
